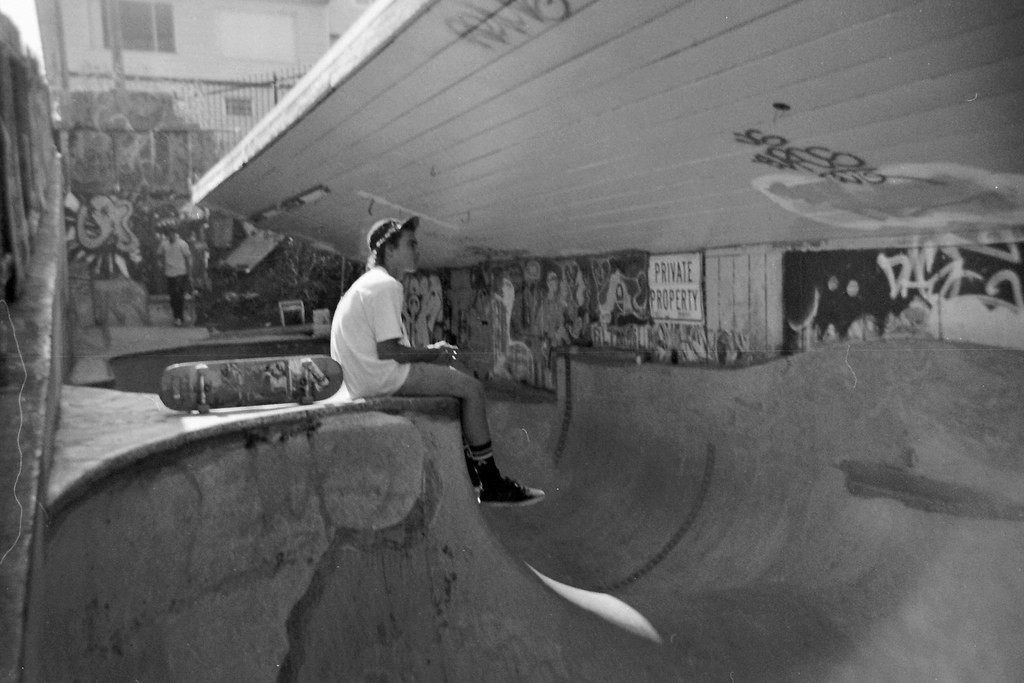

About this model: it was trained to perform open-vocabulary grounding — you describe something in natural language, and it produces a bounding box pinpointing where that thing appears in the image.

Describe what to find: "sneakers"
[469,475,545,507]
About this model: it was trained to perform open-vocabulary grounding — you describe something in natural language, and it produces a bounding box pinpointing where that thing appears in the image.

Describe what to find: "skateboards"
[158,354,344,414]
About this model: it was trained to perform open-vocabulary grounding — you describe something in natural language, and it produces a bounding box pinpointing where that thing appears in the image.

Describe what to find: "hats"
[366,215,419,252]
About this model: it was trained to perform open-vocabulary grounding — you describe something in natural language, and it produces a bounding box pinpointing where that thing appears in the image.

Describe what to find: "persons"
[324,217,546,508]
[154,223,194,327]
